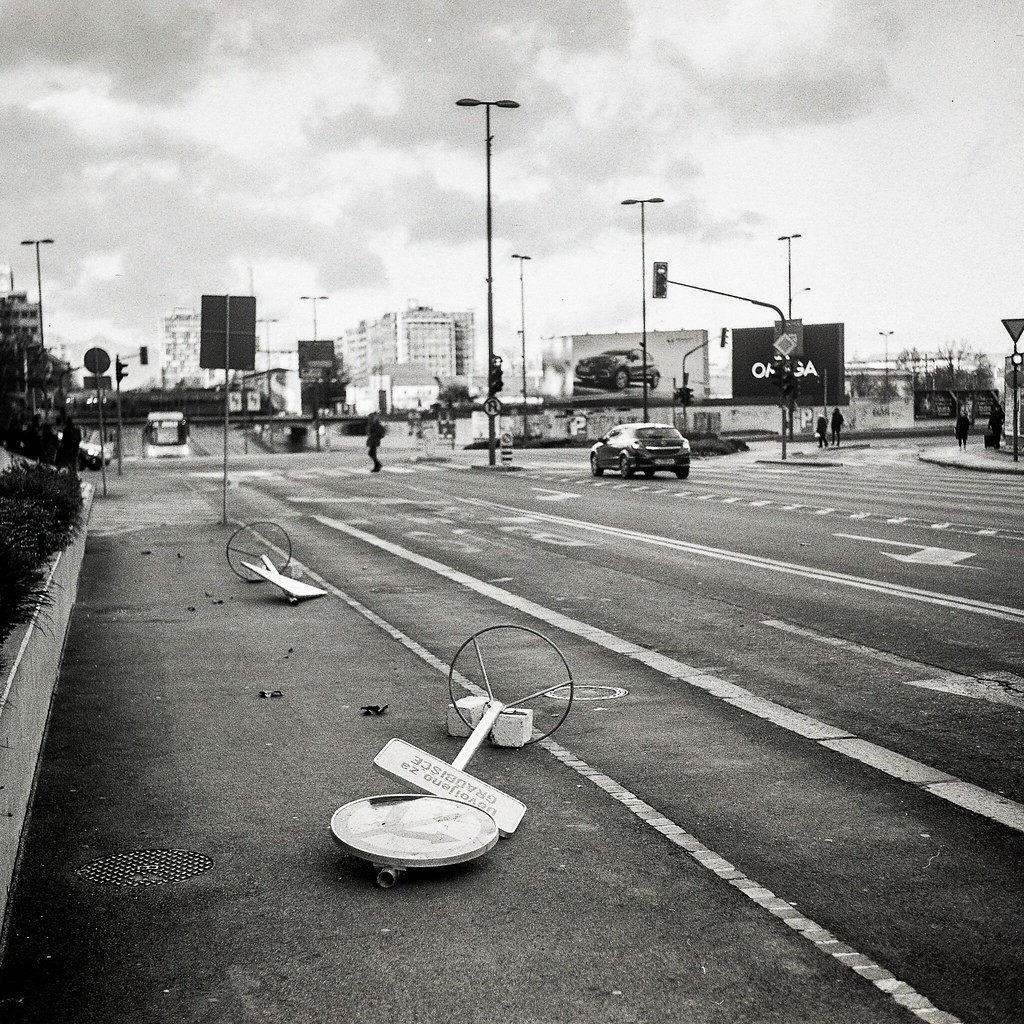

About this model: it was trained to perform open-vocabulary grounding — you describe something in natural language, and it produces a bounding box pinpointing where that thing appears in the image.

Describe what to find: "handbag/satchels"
[814,431,821,438]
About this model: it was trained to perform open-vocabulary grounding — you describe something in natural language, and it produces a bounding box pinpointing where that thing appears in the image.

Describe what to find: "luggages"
[984,427,995,448]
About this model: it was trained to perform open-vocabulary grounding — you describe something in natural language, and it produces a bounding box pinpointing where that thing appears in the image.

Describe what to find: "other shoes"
[372,462,382,472]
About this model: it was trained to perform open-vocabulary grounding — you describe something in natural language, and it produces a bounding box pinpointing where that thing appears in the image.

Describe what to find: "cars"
[588,422,691,479]
[574,347,660,391]
[52,432,110,472]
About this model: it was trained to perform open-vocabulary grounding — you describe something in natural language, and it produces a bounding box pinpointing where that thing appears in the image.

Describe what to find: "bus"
[141,409,190,458]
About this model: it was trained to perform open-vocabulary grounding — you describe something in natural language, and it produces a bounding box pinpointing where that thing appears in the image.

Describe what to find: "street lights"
[879,331,895,395]
[301,296,330,451]
[21,239,55,420]
[256,319,280,454]
[456,98,520,468]
[511,254,532,450]
[779,234,811,442]
[621,197,664,424]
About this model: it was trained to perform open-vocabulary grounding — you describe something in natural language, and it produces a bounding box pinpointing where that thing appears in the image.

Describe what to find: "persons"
[831,408,844,447]
[366,416,387,473]
[988,405,1005,449]
[816,414,828,448]
[955,409,969,451]
[61,418,81,476]
[0,415,60,466]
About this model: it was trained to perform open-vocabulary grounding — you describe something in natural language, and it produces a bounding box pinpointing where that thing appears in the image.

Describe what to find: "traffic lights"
[116,361,128,383]
[721,328,729,347]
[490,368,504,392]
[1011,353,1023,366]
